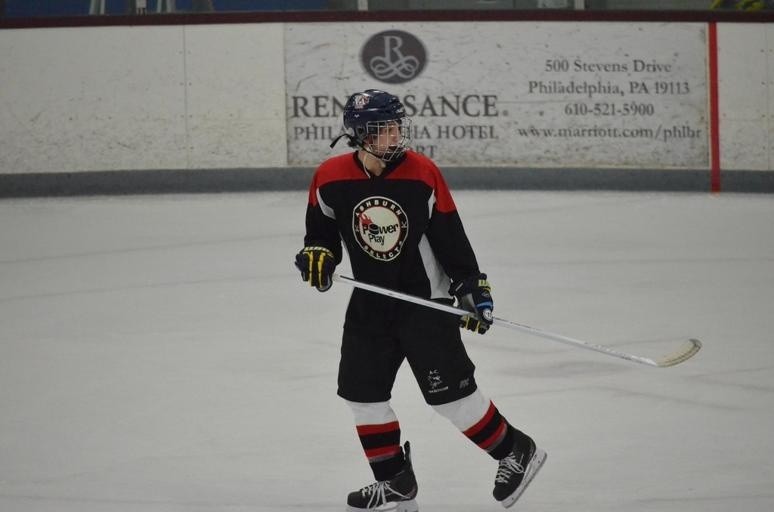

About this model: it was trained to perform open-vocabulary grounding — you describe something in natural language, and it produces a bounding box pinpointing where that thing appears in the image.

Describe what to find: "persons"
[293,89,536,508]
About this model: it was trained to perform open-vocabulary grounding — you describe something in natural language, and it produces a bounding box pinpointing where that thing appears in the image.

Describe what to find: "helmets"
[342,90,412,161]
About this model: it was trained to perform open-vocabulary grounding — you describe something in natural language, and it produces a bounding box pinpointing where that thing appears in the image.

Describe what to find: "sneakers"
[494,430,535,501]
[347,465,418,508]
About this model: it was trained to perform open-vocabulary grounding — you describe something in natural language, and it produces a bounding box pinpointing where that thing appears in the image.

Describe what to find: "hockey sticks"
[331,274,702,367]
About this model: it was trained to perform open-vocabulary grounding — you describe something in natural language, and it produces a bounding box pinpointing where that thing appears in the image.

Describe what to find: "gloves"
[298,238,335,287]
[449,272,493,334]
